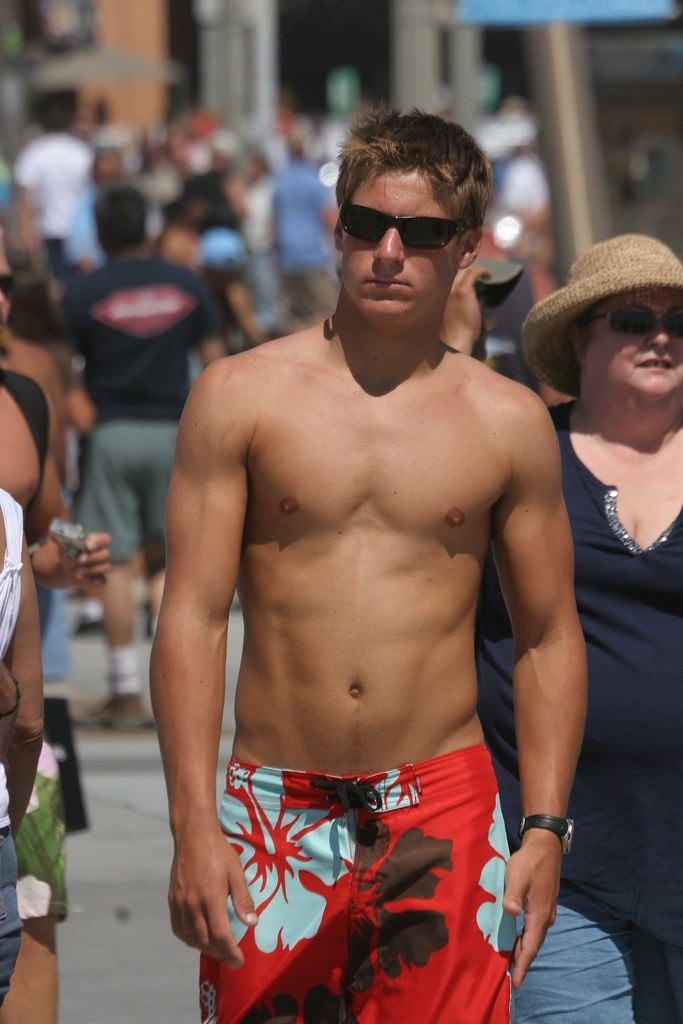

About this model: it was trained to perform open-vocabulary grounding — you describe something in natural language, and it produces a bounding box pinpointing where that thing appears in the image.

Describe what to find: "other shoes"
[89,691,153,732]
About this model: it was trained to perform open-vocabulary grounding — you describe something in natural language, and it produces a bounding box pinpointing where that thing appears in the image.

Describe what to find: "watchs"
[518,814,574,856]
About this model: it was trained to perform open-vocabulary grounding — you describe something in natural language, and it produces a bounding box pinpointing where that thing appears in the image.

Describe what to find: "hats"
[197,226,244,271]
[521,235,683,398]
[465,257,524,308]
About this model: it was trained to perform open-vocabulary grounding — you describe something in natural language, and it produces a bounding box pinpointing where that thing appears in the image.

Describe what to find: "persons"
[147,113,590,1024]
[0,492,44,1019]
[472,233,683,1023]
[1,77,565,1023]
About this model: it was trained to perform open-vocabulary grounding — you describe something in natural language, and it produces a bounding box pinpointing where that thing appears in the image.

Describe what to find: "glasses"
[582,305,683,335]
[339,202,464,251]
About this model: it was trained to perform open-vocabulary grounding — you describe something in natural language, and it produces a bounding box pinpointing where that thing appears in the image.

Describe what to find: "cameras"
[50,521,89,560]
